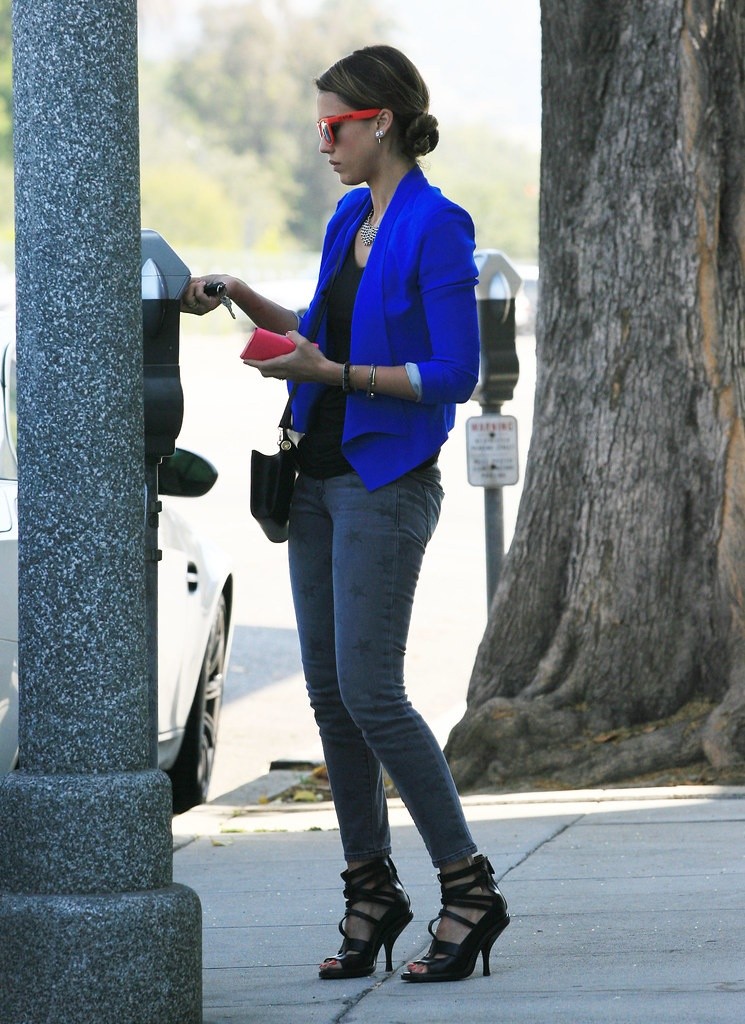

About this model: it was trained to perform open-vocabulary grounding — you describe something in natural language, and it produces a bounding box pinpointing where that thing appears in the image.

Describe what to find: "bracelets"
[340,361,377,398]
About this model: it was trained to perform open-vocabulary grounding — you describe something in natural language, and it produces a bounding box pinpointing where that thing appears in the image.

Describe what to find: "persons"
[175,43,516,982]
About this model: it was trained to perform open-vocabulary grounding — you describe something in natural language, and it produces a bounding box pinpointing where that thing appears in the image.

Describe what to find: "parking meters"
[137,233,191,461]
[475,250,521,408]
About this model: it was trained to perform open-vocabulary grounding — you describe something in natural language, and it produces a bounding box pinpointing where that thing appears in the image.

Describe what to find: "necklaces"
[360,209,382,245]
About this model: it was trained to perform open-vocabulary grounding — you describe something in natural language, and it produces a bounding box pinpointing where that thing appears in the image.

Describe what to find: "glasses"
[317,109,382,145]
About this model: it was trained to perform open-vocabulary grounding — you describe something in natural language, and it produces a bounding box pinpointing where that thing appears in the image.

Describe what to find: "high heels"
[403,858,510,983]
[318,860,413,979]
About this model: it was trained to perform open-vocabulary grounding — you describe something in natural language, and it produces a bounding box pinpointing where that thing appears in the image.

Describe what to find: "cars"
[0,343,235,817]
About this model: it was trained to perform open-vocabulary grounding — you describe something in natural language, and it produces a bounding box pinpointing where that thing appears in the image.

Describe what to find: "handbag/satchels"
[250,449,292,543]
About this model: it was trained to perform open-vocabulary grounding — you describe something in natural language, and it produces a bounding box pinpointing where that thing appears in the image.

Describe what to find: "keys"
[204,281,237,318]
